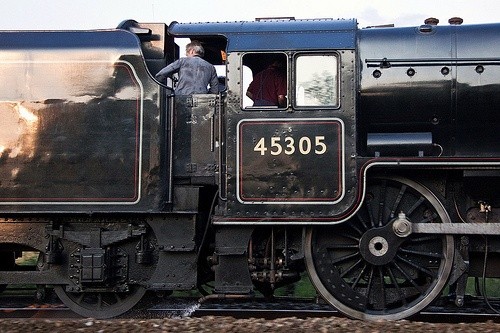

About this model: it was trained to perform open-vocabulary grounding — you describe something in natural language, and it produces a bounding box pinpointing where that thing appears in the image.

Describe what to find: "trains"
[0,14,500,322]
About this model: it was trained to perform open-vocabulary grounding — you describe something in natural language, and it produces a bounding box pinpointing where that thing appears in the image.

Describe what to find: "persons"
[155,40,220,96]
[246,55,287,108]
